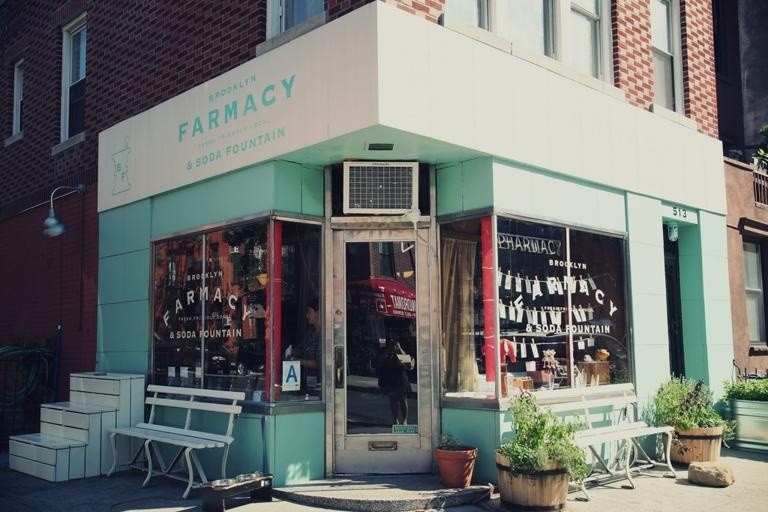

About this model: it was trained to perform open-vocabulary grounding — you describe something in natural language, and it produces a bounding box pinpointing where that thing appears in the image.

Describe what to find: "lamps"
[42,182,88,240]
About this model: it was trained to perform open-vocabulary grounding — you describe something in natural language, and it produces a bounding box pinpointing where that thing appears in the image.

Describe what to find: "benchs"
[104,382,246,500]
[525,382,678,500]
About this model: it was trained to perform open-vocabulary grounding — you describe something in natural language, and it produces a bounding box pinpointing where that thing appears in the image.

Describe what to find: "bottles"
[501,364,509,398]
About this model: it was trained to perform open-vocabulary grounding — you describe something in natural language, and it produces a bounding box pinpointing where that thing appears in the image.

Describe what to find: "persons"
[279,296,322,397]
[373,334,414,427]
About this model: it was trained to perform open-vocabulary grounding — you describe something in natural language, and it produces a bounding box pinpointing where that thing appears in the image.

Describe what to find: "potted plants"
[493,388,589,511]
[435,431,480,488]
[652,370,768,469]
